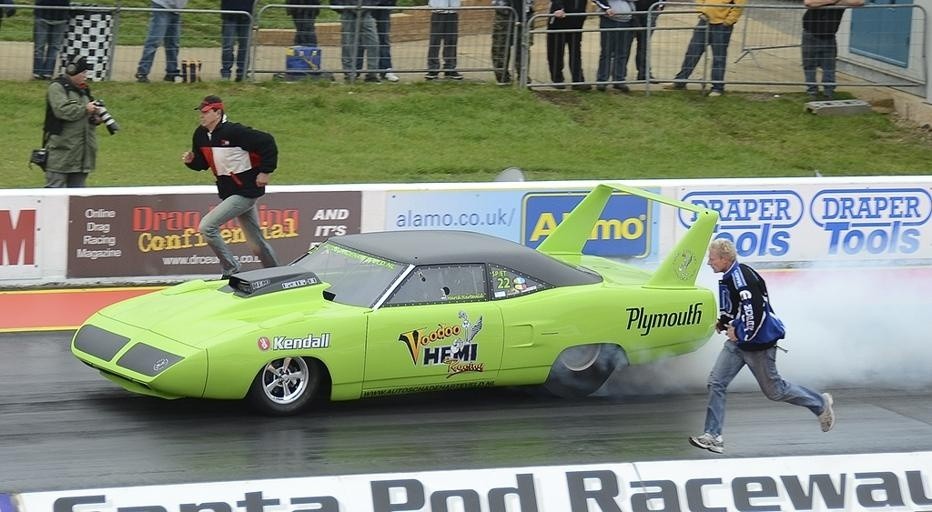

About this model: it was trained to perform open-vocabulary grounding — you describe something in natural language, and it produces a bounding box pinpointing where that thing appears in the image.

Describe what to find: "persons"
[663,0,748,97]
[356,0,400,82]
[689,238,835,455]
[624,0,665,84]
[181,95,278,280]
[284,0,317,48]
[329,0,380,83]
[801,0,865,97]
[592,0,636,92]
[134,0,183,83]
[492,0,534,85]
[424,0,464,79]
[220,0,256,83]
[41,56,102,189]
[546,0,592,89]
[32,0,69,80]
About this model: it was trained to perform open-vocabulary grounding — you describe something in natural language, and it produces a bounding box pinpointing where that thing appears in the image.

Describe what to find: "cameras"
[92,99,119,136]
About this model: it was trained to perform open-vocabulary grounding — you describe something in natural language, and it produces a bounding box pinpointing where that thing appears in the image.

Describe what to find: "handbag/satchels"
[28,80,69,170]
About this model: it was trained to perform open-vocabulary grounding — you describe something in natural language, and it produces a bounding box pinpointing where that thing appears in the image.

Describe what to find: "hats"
[193,95,224,112]
[65,55,93,75]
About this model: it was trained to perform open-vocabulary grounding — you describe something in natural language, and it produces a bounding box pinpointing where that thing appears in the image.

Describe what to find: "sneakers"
[662,83,687,90]
[708,89,723,96]
[687,432,725,455]
[596,84,606,91]
[424,73,439,79]
[823,87,835,97]
[135,73,150,82]
[444,72,464,79]
[817,392,836,433]
[637,75,659,84]
[614,84,629,92]
[553,81,566,90]
[384,68,399,82]
[163,75,184,82]
[806,88,819,95]
[571,84,592,91]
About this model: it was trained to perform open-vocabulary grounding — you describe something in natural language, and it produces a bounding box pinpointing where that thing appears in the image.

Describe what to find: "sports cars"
[72,184,717,414]
